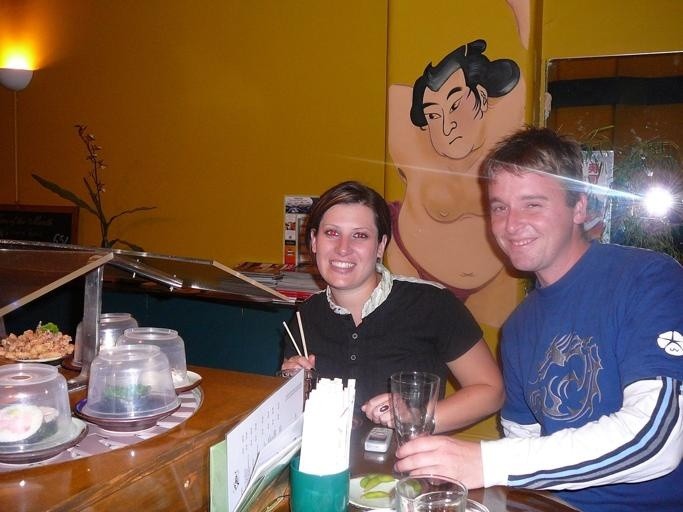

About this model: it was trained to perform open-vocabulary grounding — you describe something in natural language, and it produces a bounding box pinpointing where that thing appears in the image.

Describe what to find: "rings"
[378,402,389,412]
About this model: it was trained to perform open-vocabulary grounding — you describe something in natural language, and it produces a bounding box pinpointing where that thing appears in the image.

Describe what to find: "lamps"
[0,68,32,93]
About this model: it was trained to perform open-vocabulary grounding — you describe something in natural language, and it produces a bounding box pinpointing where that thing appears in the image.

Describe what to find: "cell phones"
[364,426,394,453]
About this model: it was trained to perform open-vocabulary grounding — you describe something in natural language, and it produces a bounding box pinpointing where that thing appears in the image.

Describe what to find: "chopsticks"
[283,311,311,380]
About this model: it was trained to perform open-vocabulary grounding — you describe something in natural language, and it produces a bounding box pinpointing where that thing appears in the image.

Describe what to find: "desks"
[0,249,326,376]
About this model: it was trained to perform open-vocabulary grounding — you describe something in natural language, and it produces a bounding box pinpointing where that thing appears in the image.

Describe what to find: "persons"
[273,179,507,435]
[392,124,683,512]
[385,38,526,332]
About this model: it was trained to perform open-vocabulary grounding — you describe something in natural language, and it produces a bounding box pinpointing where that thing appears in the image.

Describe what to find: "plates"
[7,340,71,366]
[349,474,414,509]
[0,416,89,464]
[105,367,203,395]
[75,390,181,433]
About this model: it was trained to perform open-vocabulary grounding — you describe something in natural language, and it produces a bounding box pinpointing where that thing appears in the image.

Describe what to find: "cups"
[390,370,441,448]
[395,473,468,512]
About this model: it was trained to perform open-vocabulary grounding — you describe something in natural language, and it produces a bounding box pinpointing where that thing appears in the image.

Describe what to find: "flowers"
[33,121,155,253]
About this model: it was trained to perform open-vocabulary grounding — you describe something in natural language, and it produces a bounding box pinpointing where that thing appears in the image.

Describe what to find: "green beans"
[360,474,422,499]
